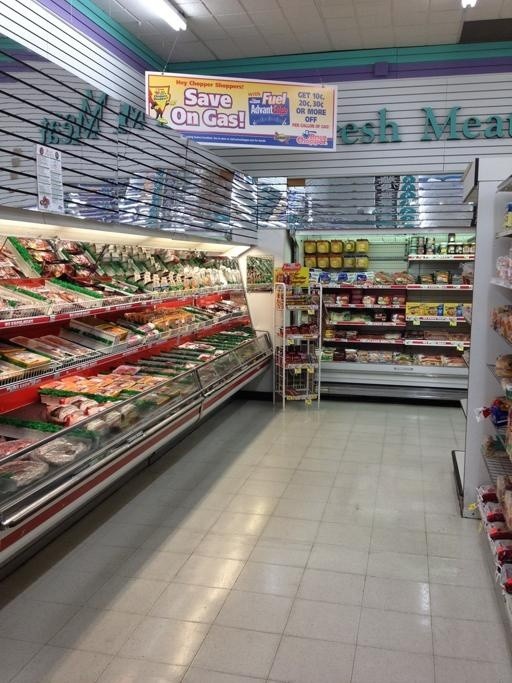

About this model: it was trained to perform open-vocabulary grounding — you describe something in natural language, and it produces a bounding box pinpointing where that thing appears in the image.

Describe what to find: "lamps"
[140,0,189,33]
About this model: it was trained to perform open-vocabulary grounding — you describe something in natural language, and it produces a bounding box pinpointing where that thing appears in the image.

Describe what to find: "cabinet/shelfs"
[401,250,471,387]
[169,230,274,428]
[297,269,405,381]
[452,153,512,626]
[270,280,324,410]
[0,226,202,568]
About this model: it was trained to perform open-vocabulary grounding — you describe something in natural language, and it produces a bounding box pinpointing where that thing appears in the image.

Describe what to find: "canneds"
[447,243,476,254]
